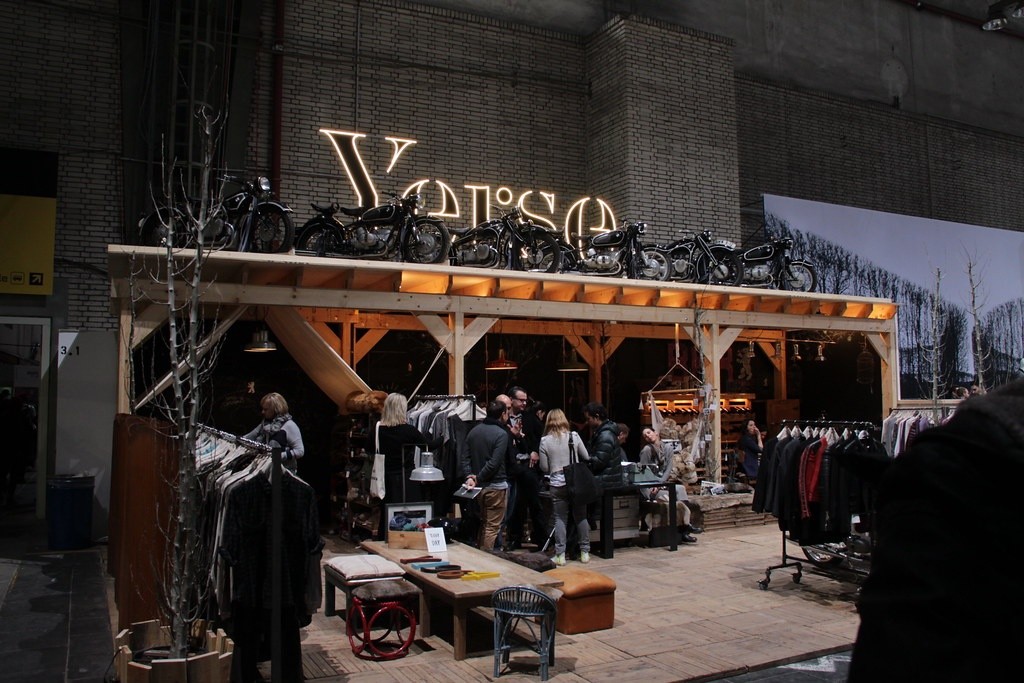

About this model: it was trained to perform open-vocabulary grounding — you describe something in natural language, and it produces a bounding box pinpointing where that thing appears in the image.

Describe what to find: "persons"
[0,390,37,495]
[496,387,623,567]
[948,381,987,398]
[737,419,764,488]
[342,391,444,541]
[846,382,1024,683]
[461,400,508,552]
[616,424,629,461]
[243,393,304,476]
[640,428,703,541]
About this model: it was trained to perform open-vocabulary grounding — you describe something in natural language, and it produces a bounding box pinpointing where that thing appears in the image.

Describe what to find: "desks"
[360,538,564,661]
[599,480,682,559]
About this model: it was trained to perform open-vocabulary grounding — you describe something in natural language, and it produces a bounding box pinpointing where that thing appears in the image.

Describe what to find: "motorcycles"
[137,172,819,295]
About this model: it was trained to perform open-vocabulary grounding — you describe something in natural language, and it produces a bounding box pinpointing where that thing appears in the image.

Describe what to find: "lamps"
[557,323,589,372]
[485,320,518,371]
[746,340,756,358]
[243,306,277,353]
[772,339,782,360]
[408,446,447,482]
[791,344,802,360]
[814,341,825,362]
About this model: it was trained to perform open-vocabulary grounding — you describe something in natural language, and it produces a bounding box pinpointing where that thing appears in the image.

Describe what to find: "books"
[454,487,482,499]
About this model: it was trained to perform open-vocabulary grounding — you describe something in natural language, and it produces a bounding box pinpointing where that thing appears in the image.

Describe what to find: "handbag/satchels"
[564,462,600,503]
[370,453,385,499]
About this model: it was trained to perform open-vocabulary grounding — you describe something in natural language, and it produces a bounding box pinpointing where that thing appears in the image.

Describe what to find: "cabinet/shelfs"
[330,410,385,545]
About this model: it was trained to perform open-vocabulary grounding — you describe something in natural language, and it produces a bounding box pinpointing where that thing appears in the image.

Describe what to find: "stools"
[492,552,556,572]
[324,555,421,661]
[534,566,616,635]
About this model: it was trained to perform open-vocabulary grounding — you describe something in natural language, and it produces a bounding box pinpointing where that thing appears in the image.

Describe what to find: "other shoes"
[503,541,515,551]
[688,526,704,534]
[492,549,501,554]
[681,534,697,542]
[581,550,590,562]
[550,552,566,565]
[494,545,505,552]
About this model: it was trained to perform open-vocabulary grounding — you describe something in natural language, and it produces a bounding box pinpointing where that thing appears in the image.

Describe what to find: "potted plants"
[114,102,240,683]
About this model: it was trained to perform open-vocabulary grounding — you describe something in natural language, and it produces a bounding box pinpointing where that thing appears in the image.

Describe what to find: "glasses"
[513,398,528,403]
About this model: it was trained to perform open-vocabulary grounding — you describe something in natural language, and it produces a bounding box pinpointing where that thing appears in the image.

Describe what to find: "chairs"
[490,586,557,682]
[733,449,757,485]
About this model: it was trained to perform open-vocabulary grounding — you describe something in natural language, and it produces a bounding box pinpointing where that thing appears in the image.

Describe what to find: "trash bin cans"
[47,475,95,550]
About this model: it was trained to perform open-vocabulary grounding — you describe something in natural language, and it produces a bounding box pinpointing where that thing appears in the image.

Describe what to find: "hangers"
[783,419,874,448]
[196,424,285,474]
[415,394,487,415]
